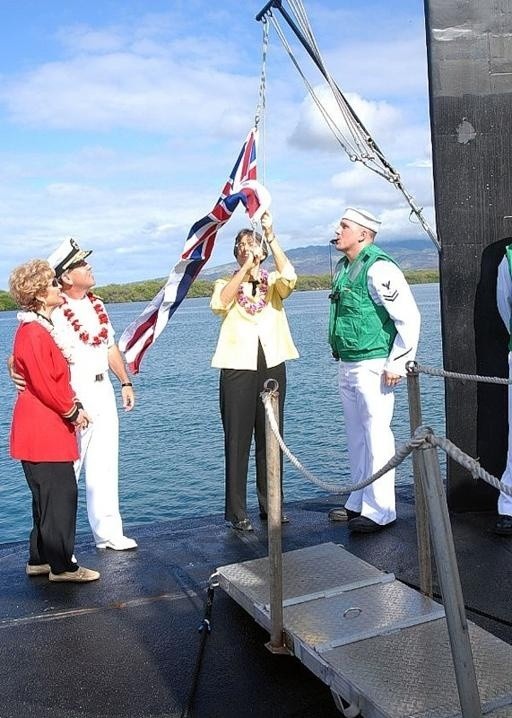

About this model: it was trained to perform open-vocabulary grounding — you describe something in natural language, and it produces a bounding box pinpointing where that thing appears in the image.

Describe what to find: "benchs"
[116,126,271,375]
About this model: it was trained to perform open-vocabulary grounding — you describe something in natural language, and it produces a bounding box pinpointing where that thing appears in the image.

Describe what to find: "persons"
[498,245,512,531]
[10,237,139,551]
[328,205,421,532]
[209,210,300,531]
[10,259,99,582]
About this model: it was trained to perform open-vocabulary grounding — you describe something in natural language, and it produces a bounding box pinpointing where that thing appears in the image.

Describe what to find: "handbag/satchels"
[267,233,275,244]
[122,383,133,387]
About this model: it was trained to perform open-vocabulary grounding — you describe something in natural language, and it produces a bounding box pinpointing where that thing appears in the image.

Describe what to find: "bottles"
[341,207,382,233]
[47,236,93,279]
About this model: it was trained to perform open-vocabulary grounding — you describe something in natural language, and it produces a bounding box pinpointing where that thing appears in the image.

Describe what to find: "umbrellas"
[96,536,138,551]
[348,516,398,533]
[260,511,290,522]
[229,519,253,531]
[328,507,361,521]
[494,515,512,535]
[26,553,101,581]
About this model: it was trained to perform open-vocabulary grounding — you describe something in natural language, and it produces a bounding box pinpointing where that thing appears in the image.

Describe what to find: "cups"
[52,279,58,287]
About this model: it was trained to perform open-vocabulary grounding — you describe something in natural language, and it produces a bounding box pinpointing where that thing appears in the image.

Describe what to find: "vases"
[16,308,77,368]
[232,265,269,316]
[56,283,111,347]
